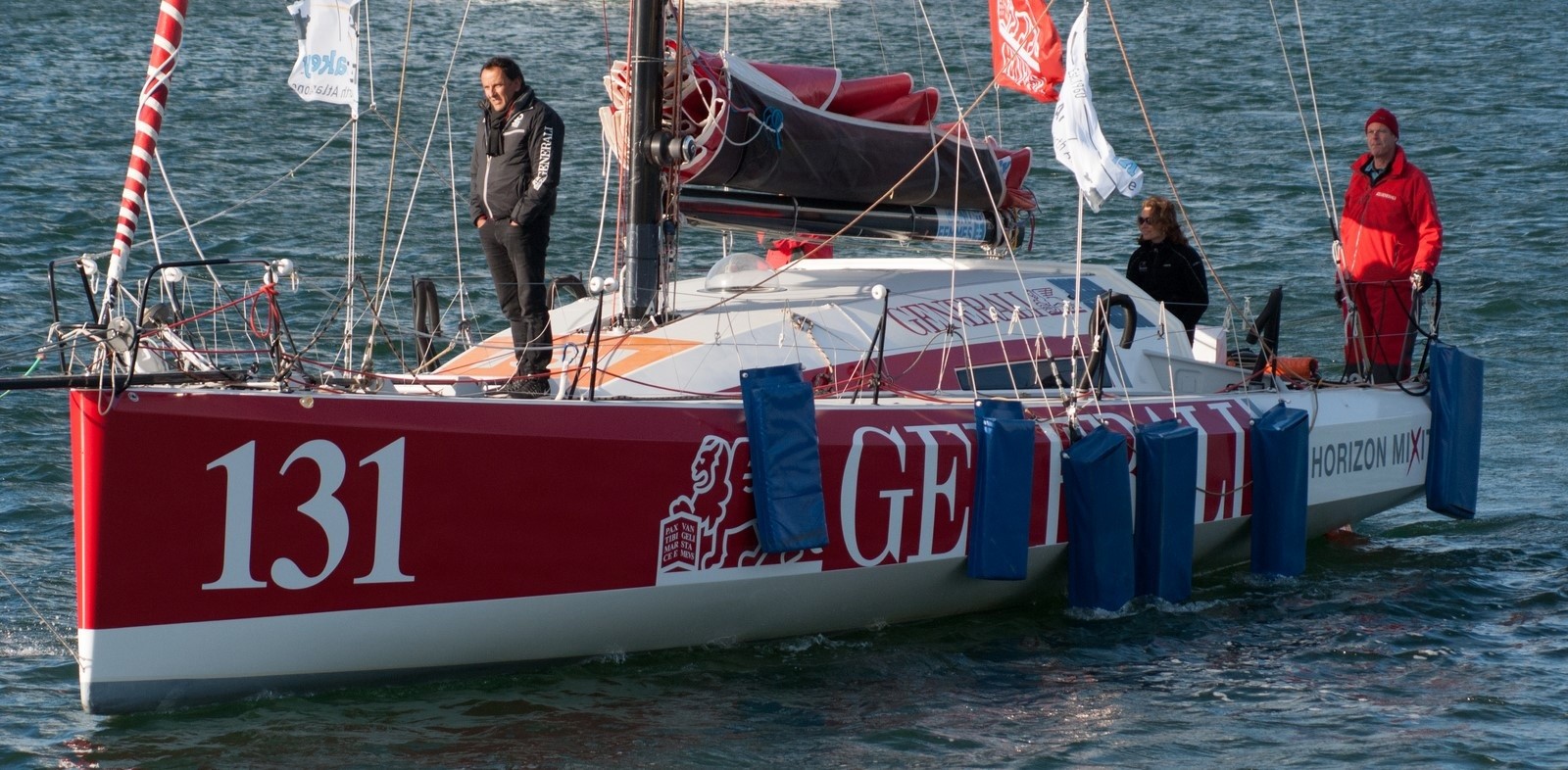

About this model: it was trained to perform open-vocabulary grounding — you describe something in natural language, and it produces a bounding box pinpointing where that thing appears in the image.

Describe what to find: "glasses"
[1137,217,1153,226]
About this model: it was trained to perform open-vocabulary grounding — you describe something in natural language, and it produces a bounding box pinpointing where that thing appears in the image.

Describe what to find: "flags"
[285,0,359,104]
[1052,5,1145,213]
[987,0,1066,103]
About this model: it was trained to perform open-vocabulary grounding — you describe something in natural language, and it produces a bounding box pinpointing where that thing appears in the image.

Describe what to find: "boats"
[0,3,1497,722]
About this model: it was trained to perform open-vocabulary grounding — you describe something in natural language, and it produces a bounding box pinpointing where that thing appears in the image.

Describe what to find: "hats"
[1365,107,1399,139]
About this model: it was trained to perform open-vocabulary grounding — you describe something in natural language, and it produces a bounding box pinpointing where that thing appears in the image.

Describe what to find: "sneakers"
[498,381,527,394]
[508,380,551,400]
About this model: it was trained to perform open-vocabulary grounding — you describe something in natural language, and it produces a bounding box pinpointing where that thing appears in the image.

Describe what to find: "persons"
[1126,197,1210,349]
[469,57,566,399]
[1335,108,1443,383]
